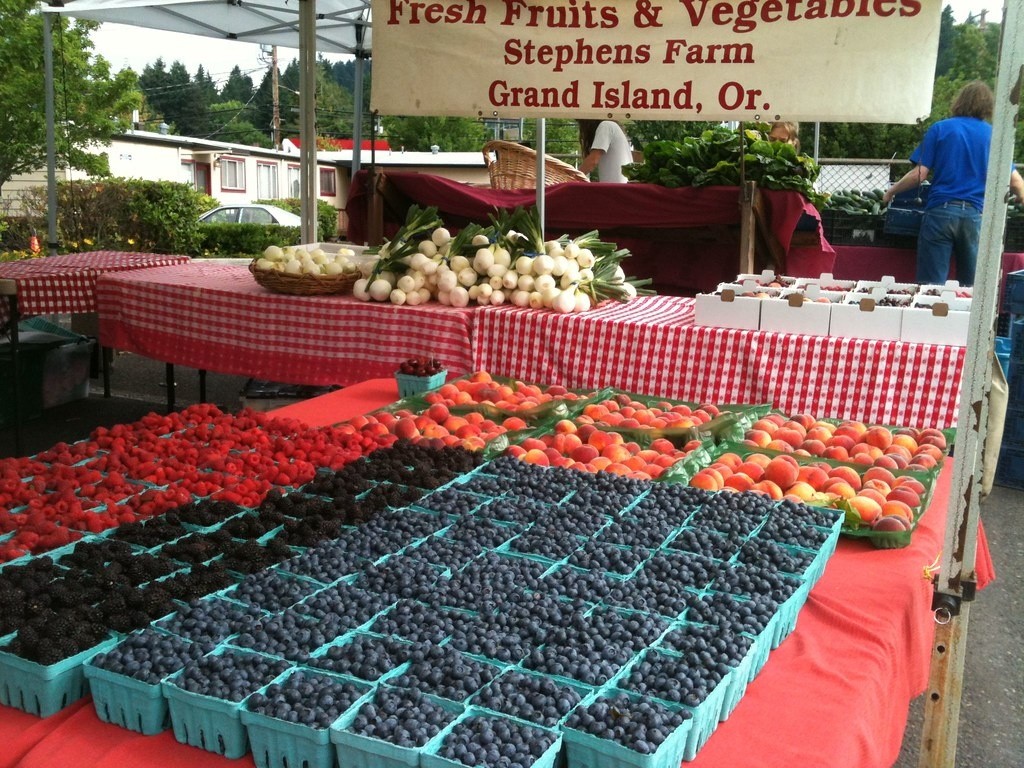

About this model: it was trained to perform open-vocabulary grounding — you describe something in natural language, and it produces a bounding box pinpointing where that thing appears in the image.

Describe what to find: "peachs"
[344,370,946,531]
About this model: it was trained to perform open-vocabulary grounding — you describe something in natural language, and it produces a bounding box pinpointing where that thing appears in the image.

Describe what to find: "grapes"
[849,293,909,317]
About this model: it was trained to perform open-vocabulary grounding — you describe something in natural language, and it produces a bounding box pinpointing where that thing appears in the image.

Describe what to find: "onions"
[354,226,596,310]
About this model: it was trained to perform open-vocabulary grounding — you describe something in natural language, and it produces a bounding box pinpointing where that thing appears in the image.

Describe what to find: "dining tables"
[1,251,190,398]
[98,264,974,432]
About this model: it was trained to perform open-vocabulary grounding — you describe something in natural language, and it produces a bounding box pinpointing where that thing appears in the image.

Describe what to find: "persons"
[575,119,635,184]
[769,122,799,149]
[882,79,1024,285]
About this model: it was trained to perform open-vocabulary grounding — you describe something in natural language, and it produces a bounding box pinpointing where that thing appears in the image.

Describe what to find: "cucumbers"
[830,179,1021,222]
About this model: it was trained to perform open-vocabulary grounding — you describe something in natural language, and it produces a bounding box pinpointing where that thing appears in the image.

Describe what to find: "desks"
[0,378,953,768]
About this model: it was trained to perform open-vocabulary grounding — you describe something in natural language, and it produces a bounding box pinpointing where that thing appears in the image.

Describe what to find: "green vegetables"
[620,126,828,205]
